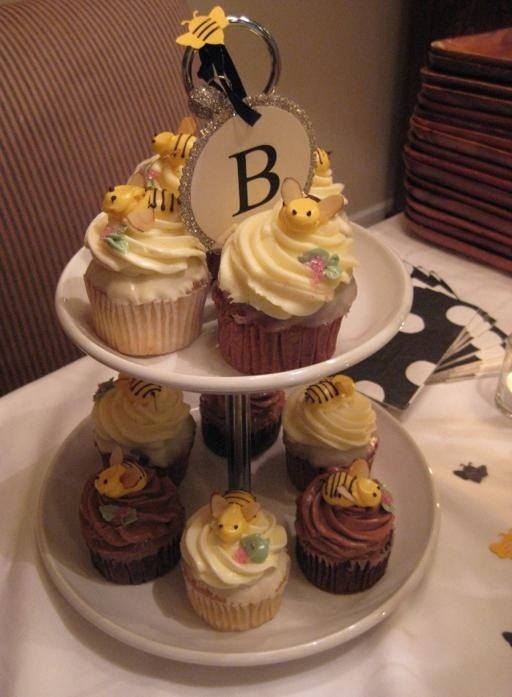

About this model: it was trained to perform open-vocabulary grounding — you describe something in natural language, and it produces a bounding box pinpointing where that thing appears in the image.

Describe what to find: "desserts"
[82,113,359,374]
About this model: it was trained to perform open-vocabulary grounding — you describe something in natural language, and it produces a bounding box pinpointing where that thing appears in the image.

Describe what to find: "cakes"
[292,456,396,594]
[88,372,196,488]
[78,446,187,584]
[281,375,381,493]
[199,390,287,459]
[177,489,292,633]
